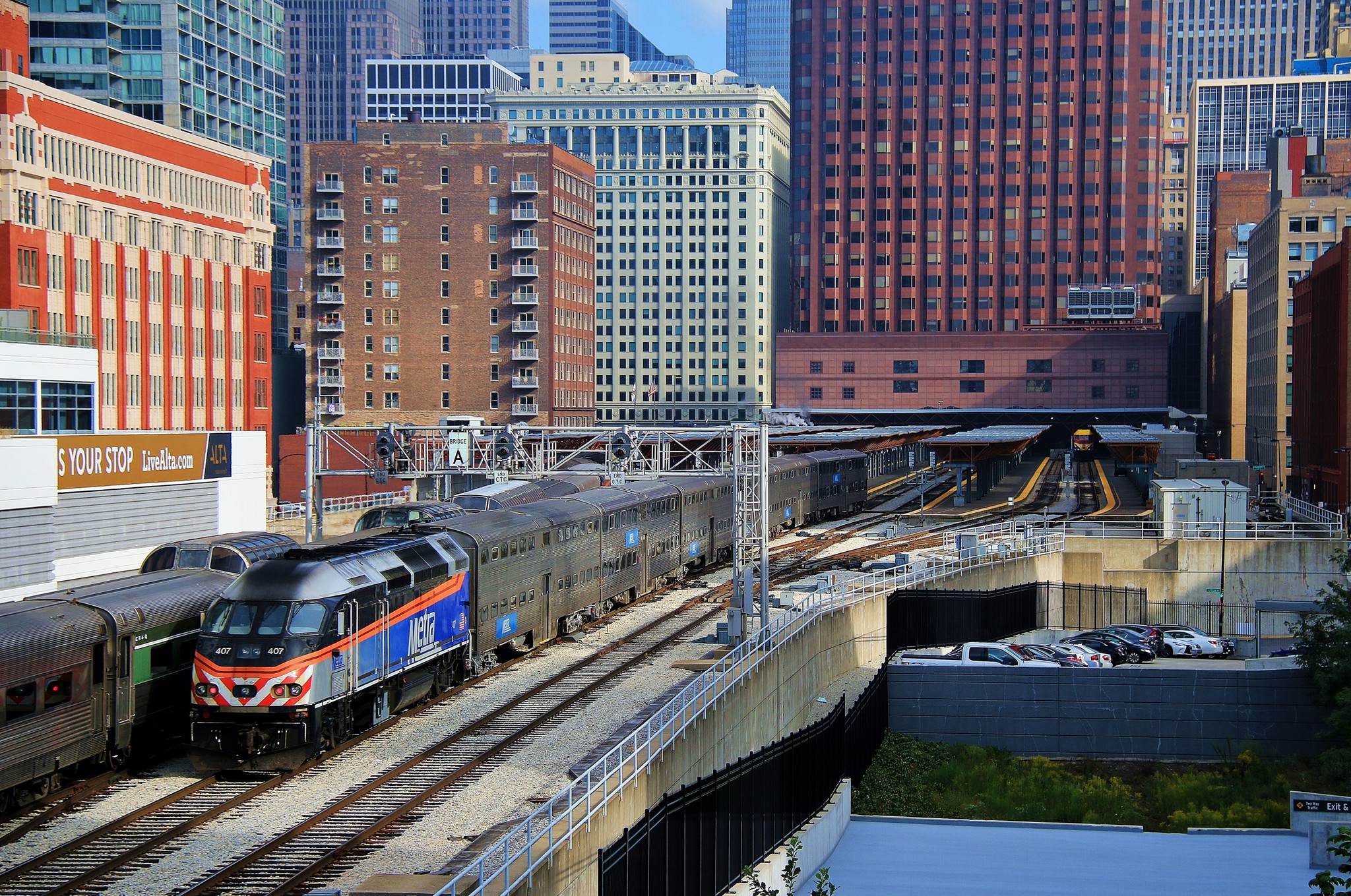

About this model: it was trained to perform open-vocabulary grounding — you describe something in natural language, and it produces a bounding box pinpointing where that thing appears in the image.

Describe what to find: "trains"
[192,450,868,768]
[1073,429,1100,461]
[1,462,610,818]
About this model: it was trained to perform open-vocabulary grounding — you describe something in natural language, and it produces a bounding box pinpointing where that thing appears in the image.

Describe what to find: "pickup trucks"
[1260,498,1280,513]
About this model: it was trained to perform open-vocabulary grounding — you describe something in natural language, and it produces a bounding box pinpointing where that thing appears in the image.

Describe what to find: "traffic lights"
[495,432,513,459]
[1259,472,1265,484]
[611,432,630,459]
[376,431,395,458]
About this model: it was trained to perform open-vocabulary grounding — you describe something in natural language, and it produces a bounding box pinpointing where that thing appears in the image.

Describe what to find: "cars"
[900,642,1113,667]
[1268,643,1348,657]
[1156,623,1236,659]
[1059,624,1164,667]
[1249,496,1257,500]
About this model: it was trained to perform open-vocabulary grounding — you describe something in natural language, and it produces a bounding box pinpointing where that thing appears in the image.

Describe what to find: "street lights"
[1252,436,1282,505]
[1231,424,1260,490]
[1219,479,1229,637]
[1333,447,1351,541]
[1216,430,1223,459]
[1270,439,1302,514]
